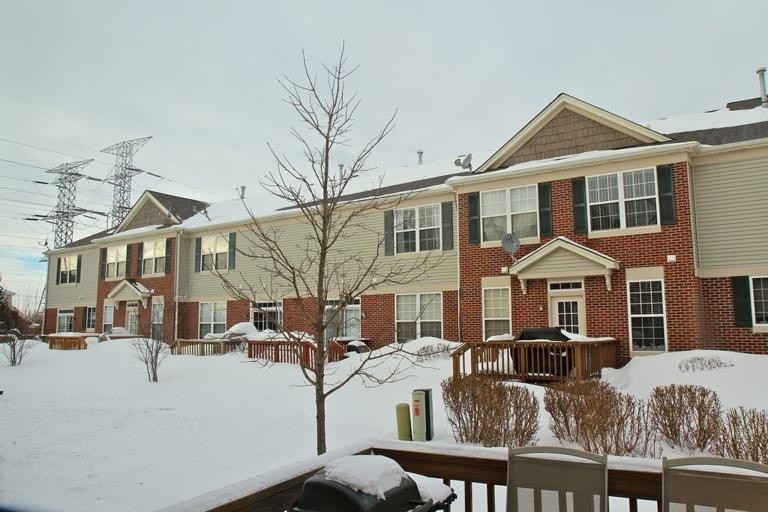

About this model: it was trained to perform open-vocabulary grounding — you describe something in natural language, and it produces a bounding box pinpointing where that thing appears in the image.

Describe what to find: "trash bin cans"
[412,388,435,442]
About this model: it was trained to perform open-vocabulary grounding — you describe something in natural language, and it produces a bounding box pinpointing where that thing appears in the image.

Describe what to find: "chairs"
[503,444,768,512]
